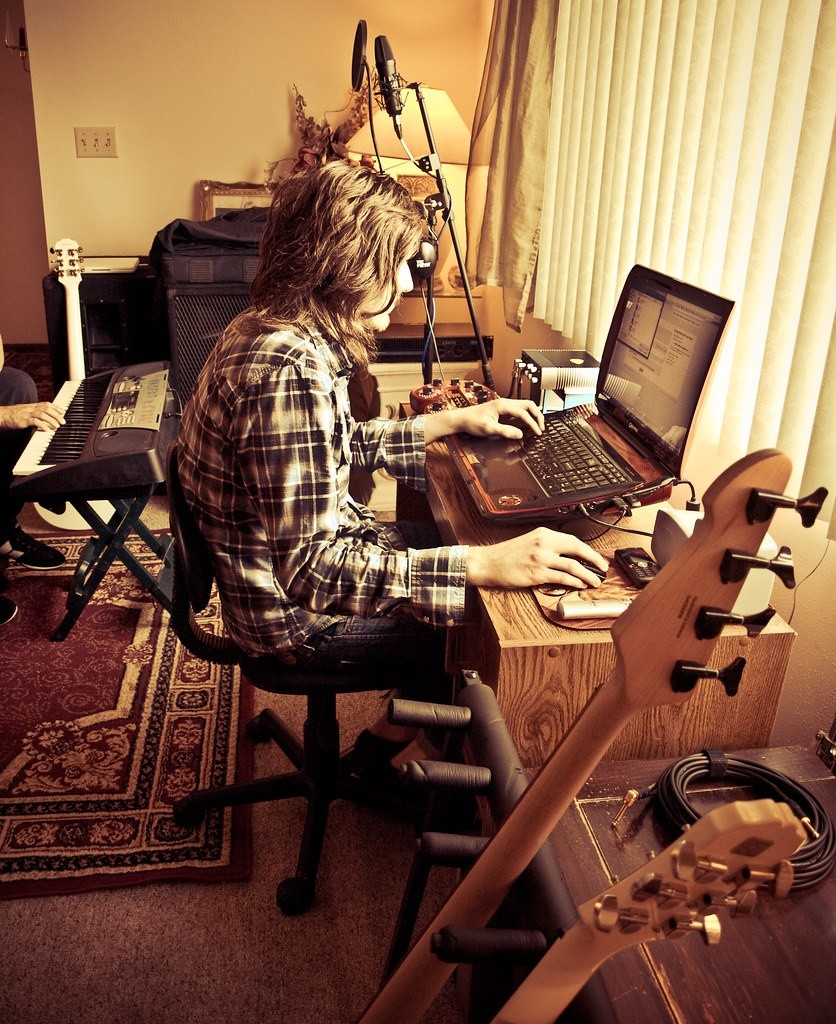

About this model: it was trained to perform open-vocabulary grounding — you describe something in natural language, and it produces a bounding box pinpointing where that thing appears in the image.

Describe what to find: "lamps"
[346,87,471,295]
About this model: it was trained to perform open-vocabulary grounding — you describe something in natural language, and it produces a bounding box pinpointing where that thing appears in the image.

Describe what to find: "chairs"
[165,442,467,916]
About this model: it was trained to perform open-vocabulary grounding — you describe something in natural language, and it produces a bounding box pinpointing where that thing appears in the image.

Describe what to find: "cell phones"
[614,547,662,588]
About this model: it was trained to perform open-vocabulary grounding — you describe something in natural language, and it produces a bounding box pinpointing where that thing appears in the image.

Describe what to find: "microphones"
[375,35,402,141]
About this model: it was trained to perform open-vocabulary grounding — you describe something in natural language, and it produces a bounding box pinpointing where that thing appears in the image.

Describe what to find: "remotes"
[558,599,633,619]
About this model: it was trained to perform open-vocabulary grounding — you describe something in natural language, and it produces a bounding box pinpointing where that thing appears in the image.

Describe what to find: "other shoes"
[0,596,19,627]
[0,523,67,568]
[334,751,446,822]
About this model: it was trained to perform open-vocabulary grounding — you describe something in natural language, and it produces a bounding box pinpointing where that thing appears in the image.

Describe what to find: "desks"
[397,403,798,770]
[525,745,836,1024]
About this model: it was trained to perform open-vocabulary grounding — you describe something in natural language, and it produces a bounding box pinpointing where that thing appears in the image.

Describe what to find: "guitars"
[428,797,809,1023]
[357,447,829,1024]
[47,238,88,381]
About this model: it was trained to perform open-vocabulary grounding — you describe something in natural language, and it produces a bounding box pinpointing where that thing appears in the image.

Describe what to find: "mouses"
[543,554,606,589]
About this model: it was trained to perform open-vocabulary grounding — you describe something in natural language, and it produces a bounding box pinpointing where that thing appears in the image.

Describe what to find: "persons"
[178,160,609,823]
[0,333,67,627]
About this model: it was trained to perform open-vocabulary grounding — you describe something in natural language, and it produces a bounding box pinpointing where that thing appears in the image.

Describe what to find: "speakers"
[168,281,254,414]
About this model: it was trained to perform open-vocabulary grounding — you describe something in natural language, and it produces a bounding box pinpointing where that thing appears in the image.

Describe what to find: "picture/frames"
[202,181,275,219]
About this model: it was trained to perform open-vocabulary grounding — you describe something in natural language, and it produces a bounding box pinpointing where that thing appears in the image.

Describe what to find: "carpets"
[0,528,254,901]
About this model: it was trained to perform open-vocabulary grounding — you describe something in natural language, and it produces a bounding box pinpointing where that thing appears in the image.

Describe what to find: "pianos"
[9,358,184,503]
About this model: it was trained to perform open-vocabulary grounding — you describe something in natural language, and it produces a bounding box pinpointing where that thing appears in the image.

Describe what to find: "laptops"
[444,263,737,519]
